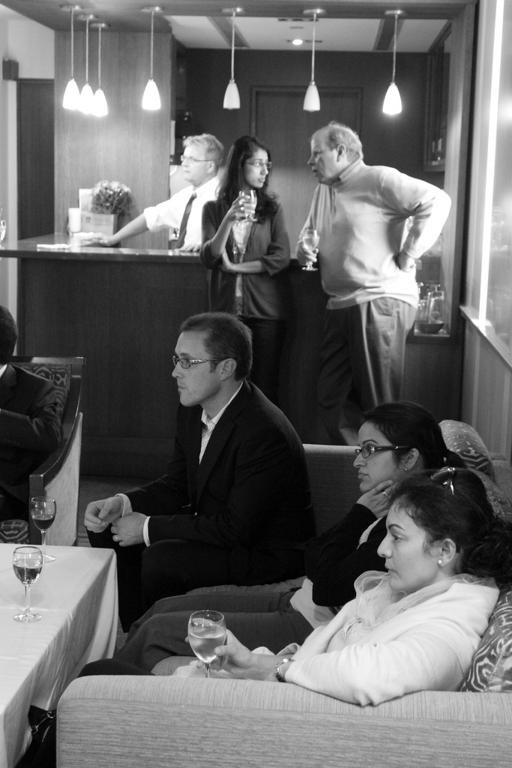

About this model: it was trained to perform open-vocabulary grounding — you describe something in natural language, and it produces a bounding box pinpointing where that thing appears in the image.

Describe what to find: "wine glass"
[301,229,320,272]
[11,545,43,625]
[187,610,228,679]
[237,190,258,222]
[29,497,57,563]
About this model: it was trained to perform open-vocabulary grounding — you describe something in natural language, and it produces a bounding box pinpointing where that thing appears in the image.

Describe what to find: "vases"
[73,212,118,244]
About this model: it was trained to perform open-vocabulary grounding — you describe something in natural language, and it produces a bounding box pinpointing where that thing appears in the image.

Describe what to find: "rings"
[382,488,392,497]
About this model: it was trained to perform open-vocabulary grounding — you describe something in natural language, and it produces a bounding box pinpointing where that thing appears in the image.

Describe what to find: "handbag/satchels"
[13,704,57,767]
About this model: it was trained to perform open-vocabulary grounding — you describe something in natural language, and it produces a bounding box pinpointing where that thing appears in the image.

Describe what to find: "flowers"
[90,179,132,215]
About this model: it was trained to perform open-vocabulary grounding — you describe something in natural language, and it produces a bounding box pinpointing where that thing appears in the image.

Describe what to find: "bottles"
[431,136,445,161]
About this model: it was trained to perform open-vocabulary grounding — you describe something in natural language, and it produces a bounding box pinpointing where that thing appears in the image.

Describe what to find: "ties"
[176,193,197,248]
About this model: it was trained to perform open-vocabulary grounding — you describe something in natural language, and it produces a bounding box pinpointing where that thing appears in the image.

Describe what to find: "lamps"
[221,5,244,110]
[381,9,405,115]
[302,7,328,113]
[137,6,163,112]
[57,3,112,118]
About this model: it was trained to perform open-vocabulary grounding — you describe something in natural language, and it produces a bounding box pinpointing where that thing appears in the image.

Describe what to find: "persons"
[297,119,453,445]
[82,310,314,633]
[0,305,64,544]
[73,132,228,253]
[27,469,512,767]
[112,398,471,670]
[199,134,294,408]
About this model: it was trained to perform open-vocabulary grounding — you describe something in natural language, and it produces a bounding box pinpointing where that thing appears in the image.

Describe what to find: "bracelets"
[273,647,296,680]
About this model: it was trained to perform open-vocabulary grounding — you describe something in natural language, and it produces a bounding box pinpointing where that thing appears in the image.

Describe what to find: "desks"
[1,539,128,768]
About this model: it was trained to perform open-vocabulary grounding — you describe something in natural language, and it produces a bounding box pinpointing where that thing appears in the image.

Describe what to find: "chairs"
[0,351,87,547]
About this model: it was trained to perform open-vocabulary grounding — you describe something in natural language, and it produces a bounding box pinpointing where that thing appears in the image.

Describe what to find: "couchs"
[43,417,507,768]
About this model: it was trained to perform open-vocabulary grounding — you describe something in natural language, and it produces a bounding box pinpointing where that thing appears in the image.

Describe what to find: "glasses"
[244,160,273,168]
[180,154,217,163]
[171,355,225,370]
[355,444,418,459]
